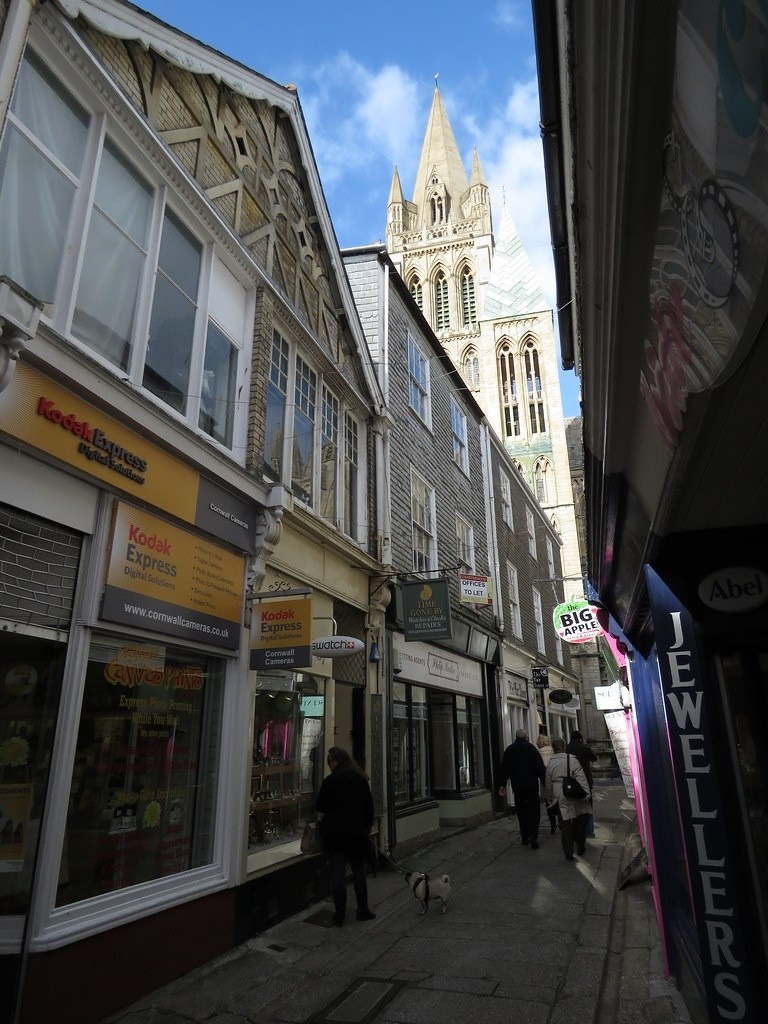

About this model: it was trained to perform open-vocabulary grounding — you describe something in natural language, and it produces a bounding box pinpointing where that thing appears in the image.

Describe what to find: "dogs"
[405,871,452,915]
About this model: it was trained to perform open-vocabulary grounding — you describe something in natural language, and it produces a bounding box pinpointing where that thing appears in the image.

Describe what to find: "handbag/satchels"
[563,777,586,799]
[301,821,324,854]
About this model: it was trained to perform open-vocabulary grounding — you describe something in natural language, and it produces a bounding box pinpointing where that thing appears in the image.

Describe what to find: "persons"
[498,728,598,861]
[314,746,376,928]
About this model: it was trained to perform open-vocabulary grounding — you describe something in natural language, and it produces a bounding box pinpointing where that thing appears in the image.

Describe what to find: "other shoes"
[522,839,528,844]
[565,853,574,859]
[356,908,376,920]
[586,833,595,839]
[333,913,343,927]
[578,848,586,856]
[532,840,539,849]
